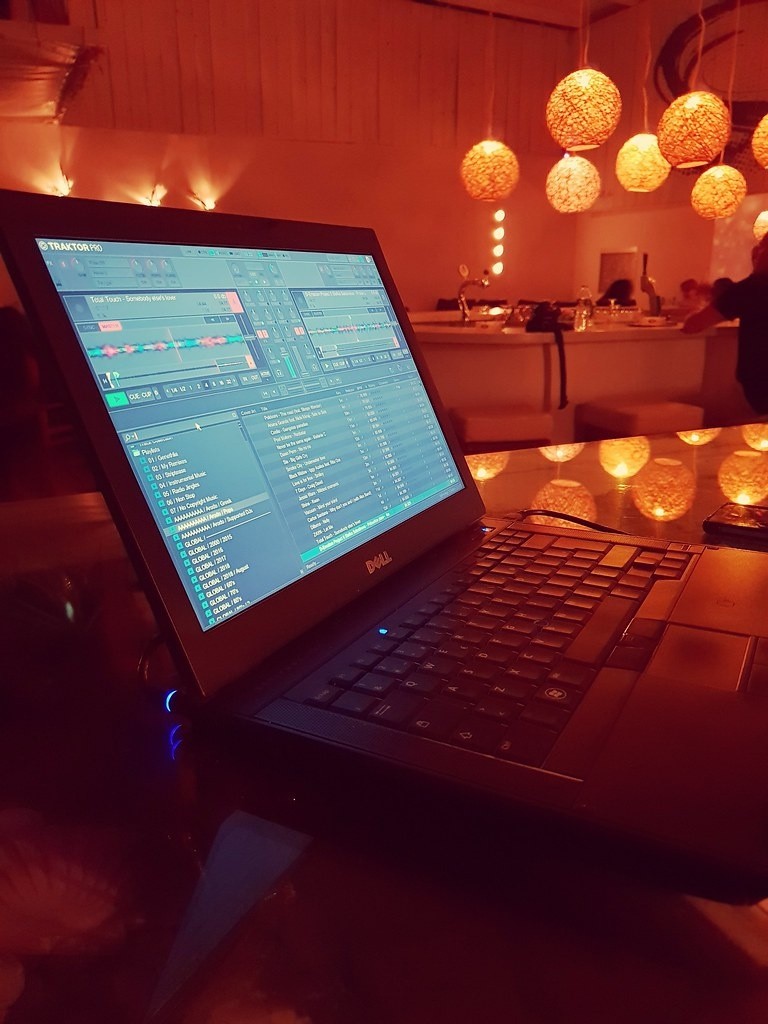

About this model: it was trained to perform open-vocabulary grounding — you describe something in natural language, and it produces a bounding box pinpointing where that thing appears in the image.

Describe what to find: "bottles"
[576,284,592,310]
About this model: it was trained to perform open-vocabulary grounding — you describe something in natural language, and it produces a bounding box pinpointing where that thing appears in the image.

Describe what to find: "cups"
[573,307,589,333]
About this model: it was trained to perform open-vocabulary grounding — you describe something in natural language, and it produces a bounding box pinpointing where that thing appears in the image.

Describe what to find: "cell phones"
[701,502,768,541]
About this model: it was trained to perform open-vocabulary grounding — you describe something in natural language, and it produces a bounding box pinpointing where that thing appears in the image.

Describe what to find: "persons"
[683,232,768,416]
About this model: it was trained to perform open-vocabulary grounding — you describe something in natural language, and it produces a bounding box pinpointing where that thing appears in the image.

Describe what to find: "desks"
[0,421,768,1024]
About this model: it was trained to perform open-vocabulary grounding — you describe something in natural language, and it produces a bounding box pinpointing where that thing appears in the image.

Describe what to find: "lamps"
[544,0,768,241]
[459,0,520,201]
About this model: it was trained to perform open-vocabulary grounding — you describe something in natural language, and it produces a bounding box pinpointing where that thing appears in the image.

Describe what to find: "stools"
[573,399,705,444]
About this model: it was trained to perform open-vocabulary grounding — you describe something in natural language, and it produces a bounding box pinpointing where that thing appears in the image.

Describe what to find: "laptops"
[0,189,768,906]
